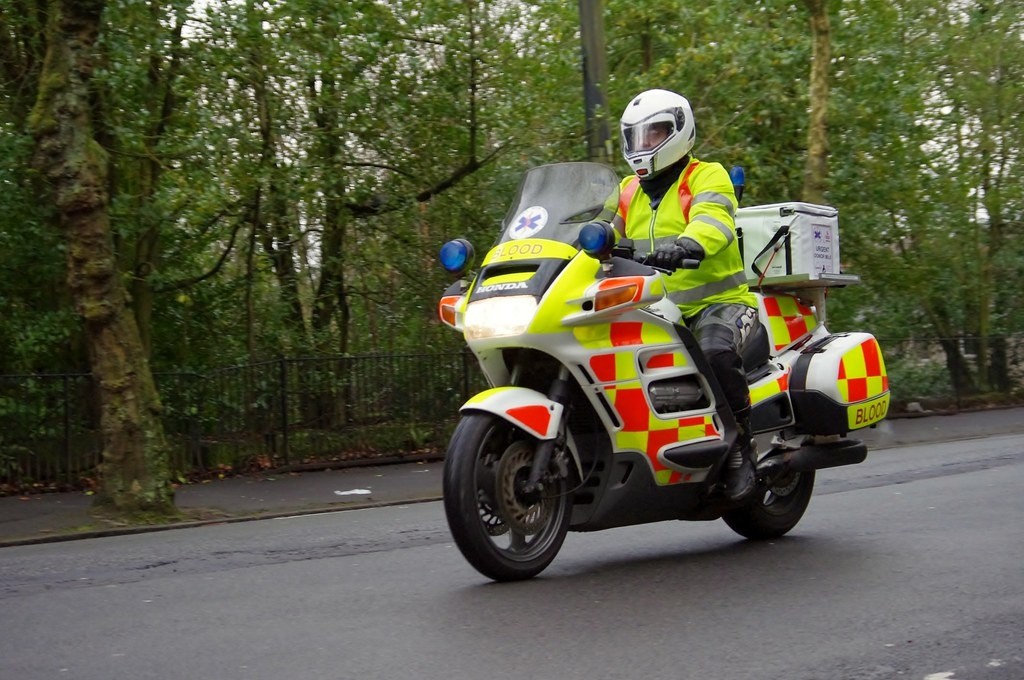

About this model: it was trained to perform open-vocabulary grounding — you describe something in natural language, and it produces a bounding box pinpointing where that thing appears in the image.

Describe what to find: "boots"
[722,406,757,500]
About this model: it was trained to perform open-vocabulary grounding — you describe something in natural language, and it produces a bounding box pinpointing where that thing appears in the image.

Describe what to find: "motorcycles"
[442,166,891,581]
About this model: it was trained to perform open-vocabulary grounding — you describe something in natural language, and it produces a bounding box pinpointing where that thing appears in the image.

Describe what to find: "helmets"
[620,90,697,180]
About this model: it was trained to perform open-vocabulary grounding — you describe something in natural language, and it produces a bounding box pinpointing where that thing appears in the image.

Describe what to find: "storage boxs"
[733,202,841,279]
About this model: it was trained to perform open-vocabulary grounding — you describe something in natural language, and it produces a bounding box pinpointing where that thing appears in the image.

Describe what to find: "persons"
[584,89,764,511]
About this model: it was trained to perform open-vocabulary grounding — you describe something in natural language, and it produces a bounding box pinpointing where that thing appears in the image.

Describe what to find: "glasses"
[629,129,667,141]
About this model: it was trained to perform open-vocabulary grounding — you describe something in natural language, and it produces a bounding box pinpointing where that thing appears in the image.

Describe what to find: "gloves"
[641,237,706,272]
